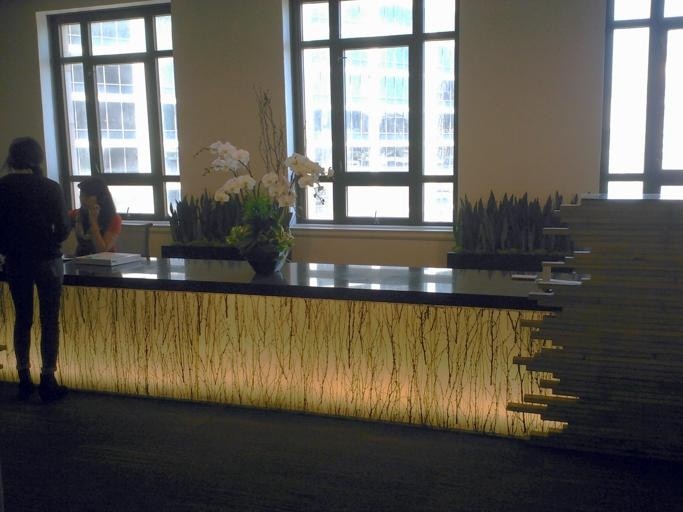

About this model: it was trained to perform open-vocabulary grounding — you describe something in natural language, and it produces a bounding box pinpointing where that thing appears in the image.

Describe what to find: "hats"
[6,136,42,165]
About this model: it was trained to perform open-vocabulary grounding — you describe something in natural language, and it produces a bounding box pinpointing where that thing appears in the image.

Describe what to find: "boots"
[17,371,38,395]
[38,374,67,401]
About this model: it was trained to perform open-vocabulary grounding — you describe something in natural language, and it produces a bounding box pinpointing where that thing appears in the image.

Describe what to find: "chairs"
[114,223,154,257]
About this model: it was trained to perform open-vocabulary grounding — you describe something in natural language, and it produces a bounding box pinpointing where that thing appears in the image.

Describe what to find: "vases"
[244,241,287,276]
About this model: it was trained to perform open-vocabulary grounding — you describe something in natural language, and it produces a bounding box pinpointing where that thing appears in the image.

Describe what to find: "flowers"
[194,84,336,259]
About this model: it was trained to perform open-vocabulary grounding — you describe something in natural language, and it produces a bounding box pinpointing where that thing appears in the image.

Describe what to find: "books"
[75,251,142,267]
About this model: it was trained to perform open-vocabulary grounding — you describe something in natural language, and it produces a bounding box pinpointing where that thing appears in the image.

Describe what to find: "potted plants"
[158,194,295,264]
[442,190,587,274]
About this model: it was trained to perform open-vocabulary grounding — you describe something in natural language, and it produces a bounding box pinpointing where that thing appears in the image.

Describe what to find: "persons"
[0,136,76,404]
[68,178,122,257]
